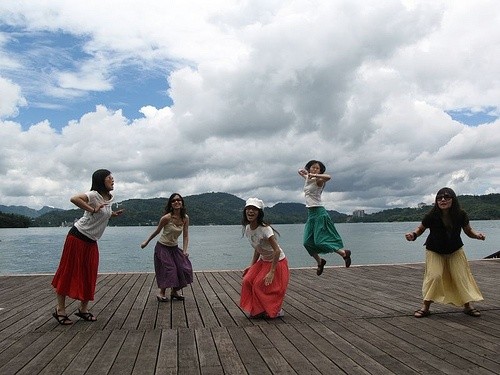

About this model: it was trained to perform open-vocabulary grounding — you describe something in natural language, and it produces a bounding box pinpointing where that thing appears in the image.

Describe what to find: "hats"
[245,198,265,210]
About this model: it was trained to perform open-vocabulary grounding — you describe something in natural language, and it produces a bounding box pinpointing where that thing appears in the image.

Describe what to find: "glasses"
[436,194,452,201]
[171,198,182,202]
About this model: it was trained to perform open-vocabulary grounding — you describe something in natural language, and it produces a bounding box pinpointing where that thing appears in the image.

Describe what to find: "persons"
[298,160,351,276]
[239,197,289,319]
[141,192,194,303]
[51,169,125,325]
[404,187,485,318]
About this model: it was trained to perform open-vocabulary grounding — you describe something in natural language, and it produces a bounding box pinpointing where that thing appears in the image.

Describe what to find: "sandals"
[53,308,73,325]
[463,307,480,317]
[414,309,430,318]
[74,308,97,321]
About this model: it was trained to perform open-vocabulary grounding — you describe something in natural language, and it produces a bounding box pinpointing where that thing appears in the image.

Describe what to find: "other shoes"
[277,308,285,316]
[317,258,326,276]
[343,250,351,268]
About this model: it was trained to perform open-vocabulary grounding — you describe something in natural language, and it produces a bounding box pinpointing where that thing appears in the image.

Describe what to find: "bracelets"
[93,208,96,214]
[413,232,417,241]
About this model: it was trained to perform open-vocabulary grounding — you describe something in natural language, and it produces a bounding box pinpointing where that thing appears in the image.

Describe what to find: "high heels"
[170,293,184,300]
[156,295,169,302]
